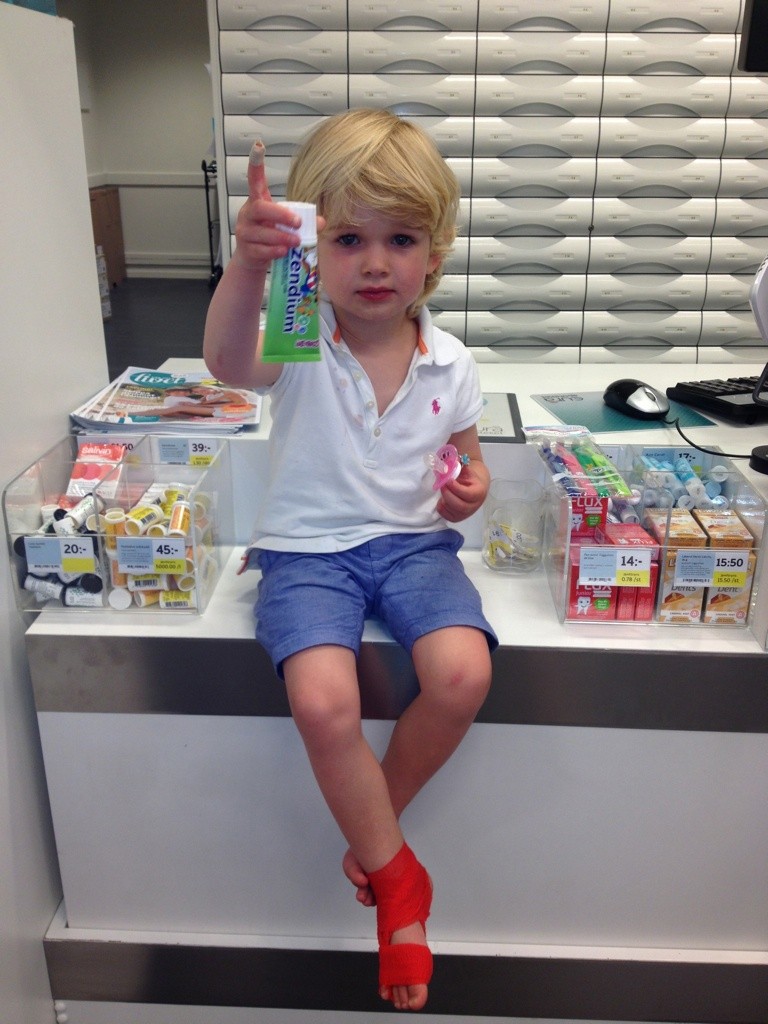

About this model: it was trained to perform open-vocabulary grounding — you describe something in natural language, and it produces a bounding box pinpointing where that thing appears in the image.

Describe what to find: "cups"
[481,479,548,573]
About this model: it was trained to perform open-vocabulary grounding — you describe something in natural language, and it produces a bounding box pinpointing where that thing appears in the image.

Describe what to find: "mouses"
[602,378,670,422]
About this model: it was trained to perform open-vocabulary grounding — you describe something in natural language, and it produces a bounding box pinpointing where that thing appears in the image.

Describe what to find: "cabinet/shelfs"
[207,0,768,368]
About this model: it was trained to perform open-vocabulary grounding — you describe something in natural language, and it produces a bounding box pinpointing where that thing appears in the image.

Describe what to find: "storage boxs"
[535,444,768,629]
[3,434,237,617]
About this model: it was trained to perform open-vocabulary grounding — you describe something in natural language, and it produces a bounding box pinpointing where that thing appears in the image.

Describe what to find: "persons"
[116,384,247,418]
[201,107,500,1011]
[165,387,200,416]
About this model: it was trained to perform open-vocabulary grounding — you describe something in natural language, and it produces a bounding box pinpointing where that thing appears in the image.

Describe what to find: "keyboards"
[665,374,768,423]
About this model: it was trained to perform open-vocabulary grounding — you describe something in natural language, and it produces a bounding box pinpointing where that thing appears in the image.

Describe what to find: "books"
[70,366,261,435]
